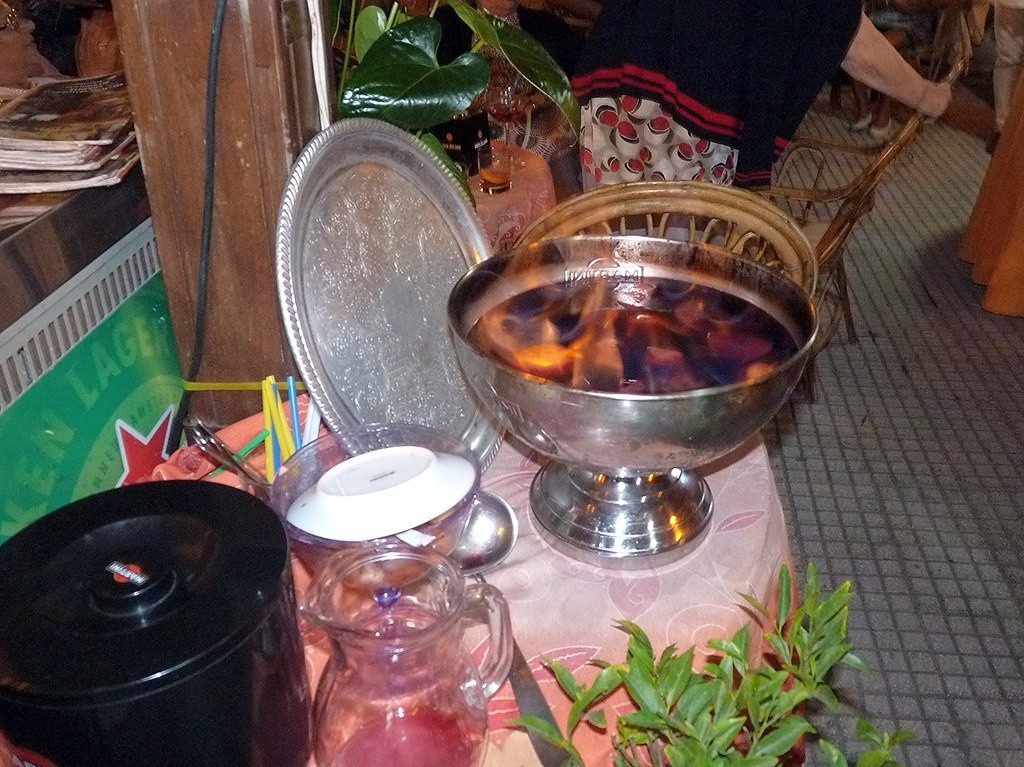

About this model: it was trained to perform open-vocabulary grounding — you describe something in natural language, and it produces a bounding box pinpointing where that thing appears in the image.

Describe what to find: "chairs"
[827,11,949,136]
[716,3,974,403]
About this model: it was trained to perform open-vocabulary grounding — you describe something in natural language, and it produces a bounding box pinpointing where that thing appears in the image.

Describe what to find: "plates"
[285,445,477,541]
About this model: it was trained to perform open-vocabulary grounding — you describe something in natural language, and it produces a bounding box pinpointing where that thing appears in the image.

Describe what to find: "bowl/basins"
[270,423,480,590]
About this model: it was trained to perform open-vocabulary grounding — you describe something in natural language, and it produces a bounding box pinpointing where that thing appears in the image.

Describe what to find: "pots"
[0,480,312,767]
[447,234,820,571]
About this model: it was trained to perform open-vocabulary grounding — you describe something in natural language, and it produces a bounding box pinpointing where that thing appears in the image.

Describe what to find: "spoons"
[449,489,574,766]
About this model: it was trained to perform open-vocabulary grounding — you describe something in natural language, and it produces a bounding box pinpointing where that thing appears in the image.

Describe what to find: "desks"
[142,386,811,766]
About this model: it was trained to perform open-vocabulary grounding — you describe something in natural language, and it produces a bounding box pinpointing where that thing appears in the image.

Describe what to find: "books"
[0,69,140,231]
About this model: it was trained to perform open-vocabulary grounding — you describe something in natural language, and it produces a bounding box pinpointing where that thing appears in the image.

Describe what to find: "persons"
[0,0,118,91]
[570,0,952,192]
[984,0,1024,154]
[849,0,940,138]
[417,0,584,177]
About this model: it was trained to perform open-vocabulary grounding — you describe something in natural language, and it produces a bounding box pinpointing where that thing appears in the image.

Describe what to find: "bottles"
[300,543,513,767]
